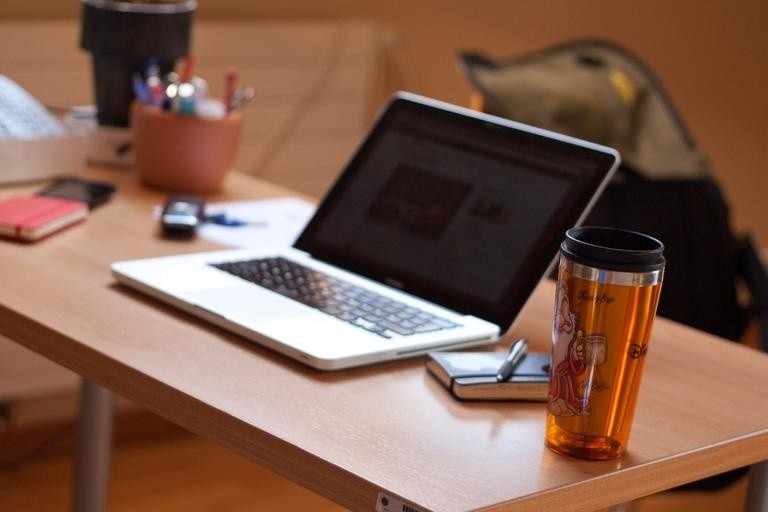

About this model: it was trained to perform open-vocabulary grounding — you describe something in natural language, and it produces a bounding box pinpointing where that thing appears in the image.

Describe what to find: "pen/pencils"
[234,87,255,108]
[203,214,246,227]
[87,158,141,172]
[225,69,238,111]
[497,338,528,382]
[133,57,225,119]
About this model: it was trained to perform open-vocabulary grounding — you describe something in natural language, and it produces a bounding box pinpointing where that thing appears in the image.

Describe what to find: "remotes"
[161,195,202,231]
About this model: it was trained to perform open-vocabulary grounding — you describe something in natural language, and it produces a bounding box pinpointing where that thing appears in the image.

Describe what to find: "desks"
[0,128,768,512]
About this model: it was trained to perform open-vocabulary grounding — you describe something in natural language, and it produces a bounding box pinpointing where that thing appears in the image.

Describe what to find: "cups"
[76,2,194,130]
[542,225,666,462]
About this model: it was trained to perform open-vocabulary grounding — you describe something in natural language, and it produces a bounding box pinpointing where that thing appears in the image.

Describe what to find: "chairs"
[461,39,767,492]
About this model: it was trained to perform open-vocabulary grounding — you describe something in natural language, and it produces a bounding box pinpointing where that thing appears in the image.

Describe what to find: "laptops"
[109,91,622,373]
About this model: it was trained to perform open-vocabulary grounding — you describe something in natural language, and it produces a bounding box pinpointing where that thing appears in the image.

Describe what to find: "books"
[426,347,551,403]
[0,196,88,246]
